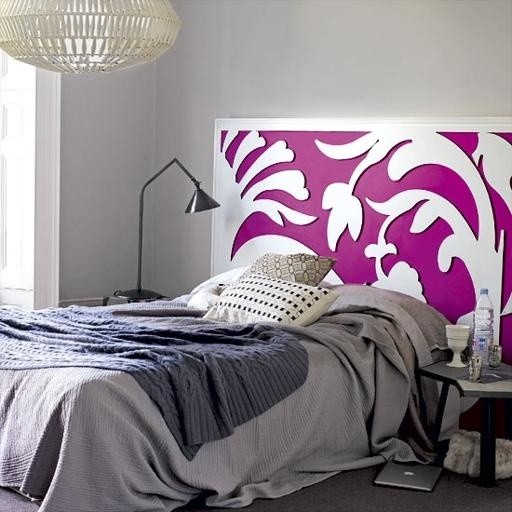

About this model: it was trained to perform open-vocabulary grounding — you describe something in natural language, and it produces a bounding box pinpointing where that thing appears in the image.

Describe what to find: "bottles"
[474,288,495,366]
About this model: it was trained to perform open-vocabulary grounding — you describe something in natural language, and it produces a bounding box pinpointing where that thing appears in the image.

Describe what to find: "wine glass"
[445,324,471,369]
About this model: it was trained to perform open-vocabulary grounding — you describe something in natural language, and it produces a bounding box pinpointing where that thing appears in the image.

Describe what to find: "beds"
[0,118,512,512]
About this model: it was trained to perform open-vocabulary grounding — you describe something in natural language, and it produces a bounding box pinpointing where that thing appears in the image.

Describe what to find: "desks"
[422,352,512,485]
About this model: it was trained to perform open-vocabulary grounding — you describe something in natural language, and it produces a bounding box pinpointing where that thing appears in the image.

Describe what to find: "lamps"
[117,156,220,298]
[1,0,182,75]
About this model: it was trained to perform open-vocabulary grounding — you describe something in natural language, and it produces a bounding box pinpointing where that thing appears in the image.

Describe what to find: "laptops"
[373,460,445,492]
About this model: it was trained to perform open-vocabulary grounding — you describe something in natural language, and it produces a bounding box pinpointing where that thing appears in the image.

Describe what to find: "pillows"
[203,272,339,328]
[246,253,337,283]
